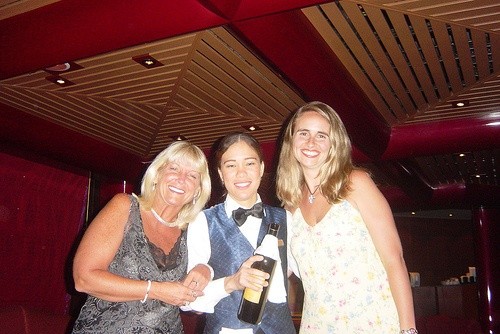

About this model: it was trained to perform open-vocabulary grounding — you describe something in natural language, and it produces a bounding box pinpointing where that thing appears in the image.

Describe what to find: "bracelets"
[198,260,215,281]
[399,326,420,334]
[140,280,152,304]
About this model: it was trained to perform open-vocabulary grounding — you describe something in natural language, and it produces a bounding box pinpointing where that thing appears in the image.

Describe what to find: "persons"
[274,101,418,334]
[178,130,296,334]
[71,137,215,334]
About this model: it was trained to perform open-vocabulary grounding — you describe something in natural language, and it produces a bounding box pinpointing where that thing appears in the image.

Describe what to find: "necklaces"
[149,207,179,228]
[303,181,322,204]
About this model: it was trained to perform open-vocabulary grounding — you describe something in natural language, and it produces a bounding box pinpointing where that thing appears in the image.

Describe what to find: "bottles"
[237,221,282,323]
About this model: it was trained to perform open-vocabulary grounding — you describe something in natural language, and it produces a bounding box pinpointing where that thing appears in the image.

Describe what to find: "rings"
[191,290,195,297]
[191,281,198,286]
[183,301,190,306]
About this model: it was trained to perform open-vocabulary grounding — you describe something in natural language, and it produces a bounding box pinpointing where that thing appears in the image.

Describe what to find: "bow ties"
[232,202,264,227]
